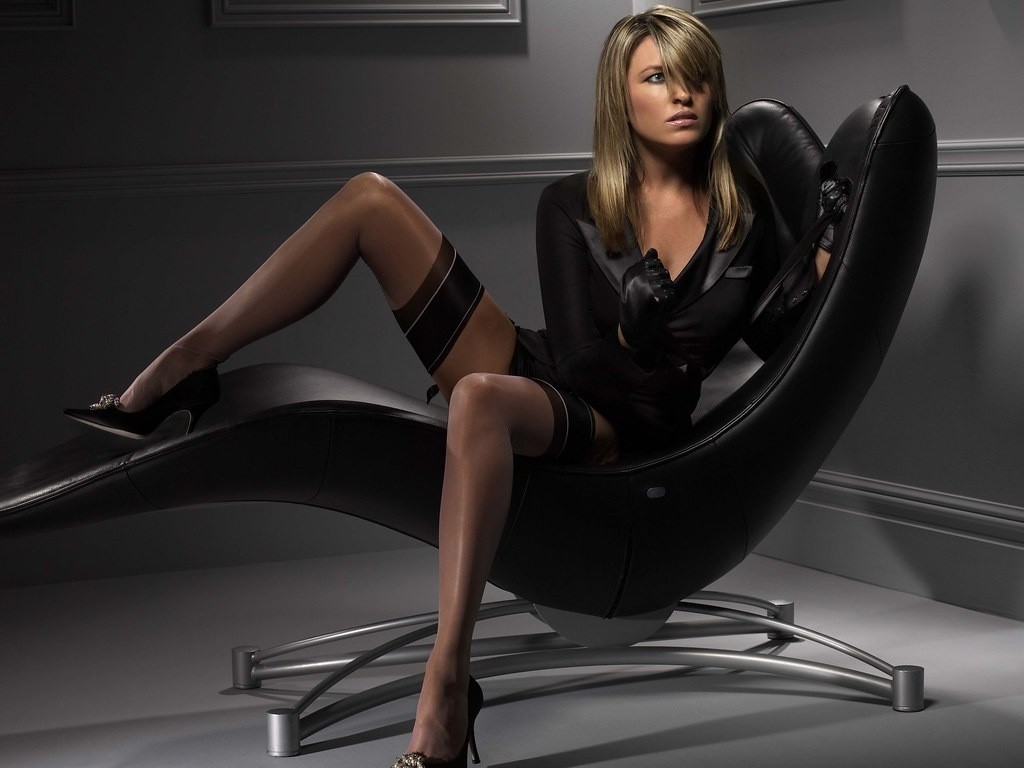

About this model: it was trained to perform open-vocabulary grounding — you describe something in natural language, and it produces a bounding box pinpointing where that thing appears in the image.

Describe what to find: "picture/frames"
[0,0,76,31]
[691,0,819,17]
[207,0,523,25]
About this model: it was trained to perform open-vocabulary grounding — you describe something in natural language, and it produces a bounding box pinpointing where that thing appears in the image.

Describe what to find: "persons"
[62,5,854,768]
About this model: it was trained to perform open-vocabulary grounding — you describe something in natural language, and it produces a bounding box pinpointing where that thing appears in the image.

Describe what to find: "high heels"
[389,675,483,768]
[60,362,221,441]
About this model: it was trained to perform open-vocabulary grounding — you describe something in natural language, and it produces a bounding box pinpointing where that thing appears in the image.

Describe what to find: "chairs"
[0,85,941,759]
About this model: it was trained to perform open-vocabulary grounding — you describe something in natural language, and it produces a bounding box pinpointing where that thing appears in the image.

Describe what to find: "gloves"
[813,178,851,255]
[619,247,674,346]
